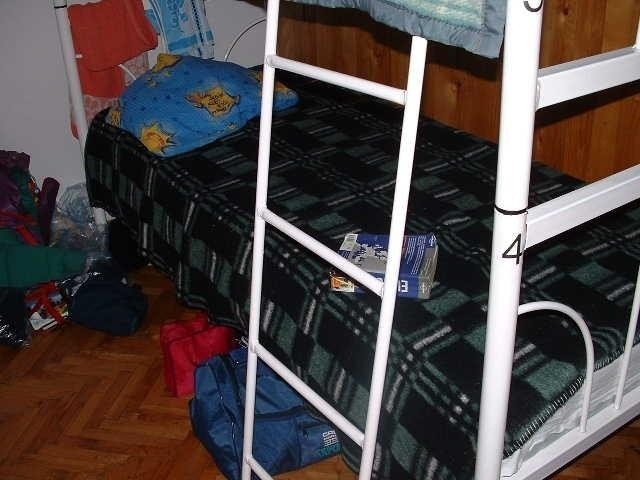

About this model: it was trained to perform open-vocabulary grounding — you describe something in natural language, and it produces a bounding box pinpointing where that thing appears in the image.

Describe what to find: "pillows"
[103,54,299,162]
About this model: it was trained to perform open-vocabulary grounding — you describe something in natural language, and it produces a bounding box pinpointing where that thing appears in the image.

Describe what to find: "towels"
[151,0,216,60]
[68,2,158,138]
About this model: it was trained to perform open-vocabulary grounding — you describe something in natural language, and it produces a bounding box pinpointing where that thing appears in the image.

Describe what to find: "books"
[328,231,440,300]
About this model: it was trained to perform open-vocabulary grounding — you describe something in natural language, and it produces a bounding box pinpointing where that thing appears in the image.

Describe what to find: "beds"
[52,1,640,480]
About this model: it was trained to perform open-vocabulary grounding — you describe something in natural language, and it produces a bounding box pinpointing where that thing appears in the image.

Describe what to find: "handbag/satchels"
[187,345,344,480]
[158,310,243,400]
